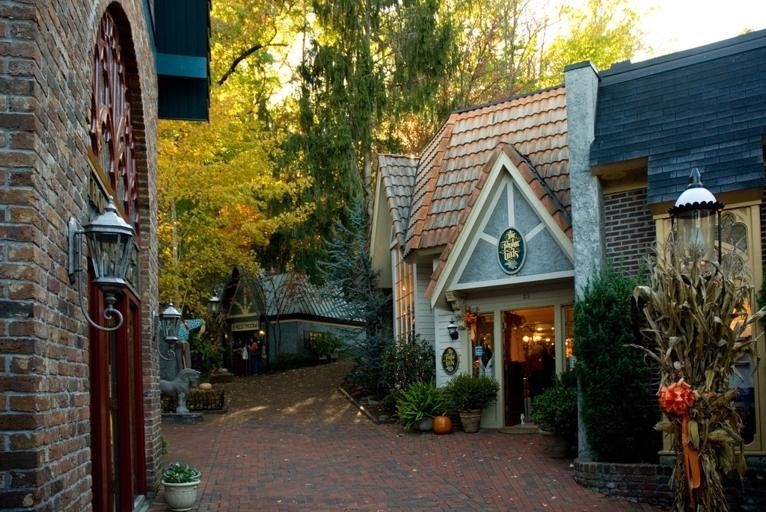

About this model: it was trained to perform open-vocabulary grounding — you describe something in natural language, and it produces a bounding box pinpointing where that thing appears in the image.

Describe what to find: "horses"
[160,367,201,415]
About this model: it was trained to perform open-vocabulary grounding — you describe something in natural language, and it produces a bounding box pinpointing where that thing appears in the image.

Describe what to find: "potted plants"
[161,461,200,512]
[396,371,501,435]
[530,383,578,458]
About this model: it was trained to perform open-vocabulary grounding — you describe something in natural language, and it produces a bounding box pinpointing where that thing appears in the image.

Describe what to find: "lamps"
[152,302,182,361]
[667,166,725,281]
[448,317,458,340]
[208,292,221,314]
[68,195,137,331]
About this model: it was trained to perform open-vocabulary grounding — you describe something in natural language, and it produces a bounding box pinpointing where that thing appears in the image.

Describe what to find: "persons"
[479,348,494,377]
[210,340,219,377]
[232,337,259,378]
